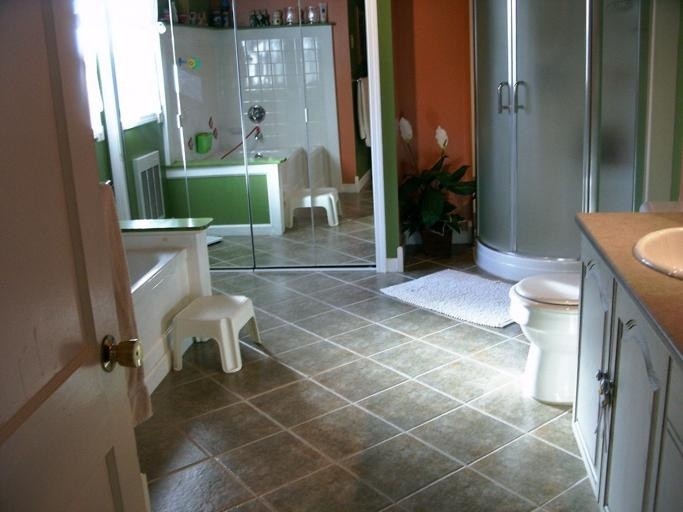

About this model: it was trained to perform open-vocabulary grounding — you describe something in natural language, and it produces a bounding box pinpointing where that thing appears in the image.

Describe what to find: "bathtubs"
[202,147,306,165]
[124,247,188,299]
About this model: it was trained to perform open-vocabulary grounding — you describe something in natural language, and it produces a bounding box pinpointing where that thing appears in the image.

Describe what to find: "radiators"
[131,150,167,219]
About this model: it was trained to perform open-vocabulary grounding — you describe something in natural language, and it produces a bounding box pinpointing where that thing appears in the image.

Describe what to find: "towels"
[95,182,152,428]
[357,77,371,146]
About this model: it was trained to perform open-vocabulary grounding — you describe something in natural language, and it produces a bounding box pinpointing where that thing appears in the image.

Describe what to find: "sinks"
[634,227,682,277]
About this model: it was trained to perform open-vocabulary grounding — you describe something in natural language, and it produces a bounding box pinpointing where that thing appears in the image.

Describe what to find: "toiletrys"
[319,3,327,22]
[308,6,315,23]
[286,6,294,25]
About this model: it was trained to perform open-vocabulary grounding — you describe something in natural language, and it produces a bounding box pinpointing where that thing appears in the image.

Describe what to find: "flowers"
[397,117,478,239]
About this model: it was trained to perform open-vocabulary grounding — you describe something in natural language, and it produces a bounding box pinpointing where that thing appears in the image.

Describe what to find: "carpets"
[381,269,519,327]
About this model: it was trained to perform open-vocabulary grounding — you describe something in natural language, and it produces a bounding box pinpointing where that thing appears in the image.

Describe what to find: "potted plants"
[177,0,191,24]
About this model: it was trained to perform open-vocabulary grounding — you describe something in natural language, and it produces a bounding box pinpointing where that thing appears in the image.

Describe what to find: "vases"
[421,230,454,260]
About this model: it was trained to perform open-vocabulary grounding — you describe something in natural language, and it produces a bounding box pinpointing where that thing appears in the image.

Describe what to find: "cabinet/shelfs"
[572,234,683,512]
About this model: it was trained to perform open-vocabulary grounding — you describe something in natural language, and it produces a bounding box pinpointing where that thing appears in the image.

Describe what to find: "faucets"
[255,134,263,141]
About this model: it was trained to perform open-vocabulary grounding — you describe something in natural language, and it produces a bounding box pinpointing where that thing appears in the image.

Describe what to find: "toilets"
[508,273,583,407]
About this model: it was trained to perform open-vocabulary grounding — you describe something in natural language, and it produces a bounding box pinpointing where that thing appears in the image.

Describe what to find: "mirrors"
[82,0,386,269]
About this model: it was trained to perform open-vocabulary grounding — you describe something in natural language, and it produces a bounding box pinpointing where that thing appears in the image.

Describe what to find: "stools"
[288,187,341,228]
[171,296,262,374]
